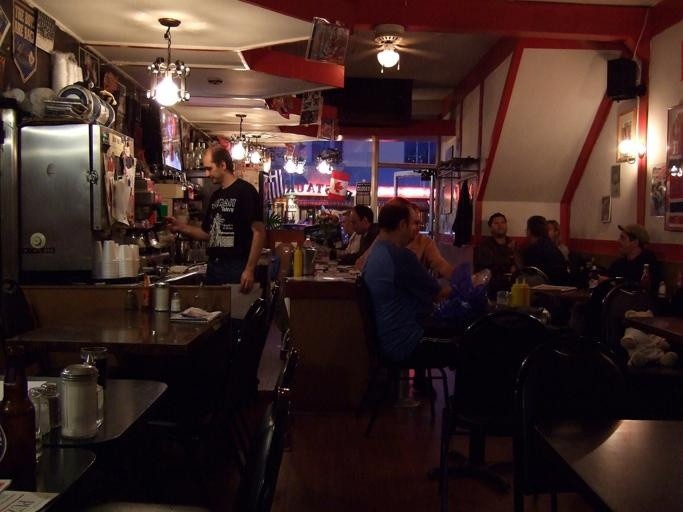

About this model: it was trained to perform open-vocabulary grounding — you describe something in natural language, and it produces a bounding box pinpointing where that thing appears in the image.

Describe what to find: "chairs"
[590,349,624,419]
[350,278,454,436]
[95,387,289,511]
[439,314,552,512]
[510,266,549,286]
[513,352,577,510]
[269,285,279,322]
[590,278,630,314]
[600,281,652,321]
[157,299,267,484]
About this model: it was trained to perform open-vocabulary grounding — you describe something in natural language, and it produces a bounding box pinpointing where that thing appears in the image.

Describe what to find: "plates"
[168,265,187,273]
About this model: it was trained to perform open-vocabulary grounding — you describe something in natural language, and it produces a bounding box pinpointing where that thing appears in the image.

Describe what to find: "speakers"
[606,57,647,101]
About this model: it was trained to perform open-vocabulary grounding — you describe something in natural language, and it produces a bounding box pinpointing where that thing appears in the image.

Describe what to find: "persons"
[472,214,525,285]
[362,202,469,423]
[608,223,663,301]
[503,216,575,290]
[337,204,380,265]
[356,194,462,302]
[336,211,361,258]
[162,144,269,295]
[548,218,572,268]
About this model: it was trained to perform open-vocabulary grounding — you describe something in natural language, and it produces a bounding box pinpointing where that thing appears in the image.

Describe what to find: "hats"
[617,220,649,246]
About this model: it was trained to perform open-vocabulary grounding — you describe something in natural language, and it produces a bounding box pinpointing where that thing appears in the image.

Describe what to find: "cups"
[114,244,119,278]
[100,241,116,280]
[130,242,140,273]
[119,246,133,276]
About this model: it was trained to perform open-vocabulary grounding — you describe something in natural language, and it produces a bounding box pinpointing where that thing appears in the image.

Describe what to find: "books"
[170,308,222,325]
[530,283,577,298]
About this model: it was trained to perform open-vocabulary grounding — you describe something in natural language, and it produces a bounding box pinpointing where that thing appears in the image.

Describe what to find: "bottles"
[181,143,189,170]
[0,345,37,495]
[142,273,151,312]
[641,263,651,296]
[195,142,201,170]
[59,364,98,439]
[125,290,136,311]
[304,235,312,249]
[201,142,206,153]
[307,209,314,225]
[188,142,195,170]
[170,292,181,312]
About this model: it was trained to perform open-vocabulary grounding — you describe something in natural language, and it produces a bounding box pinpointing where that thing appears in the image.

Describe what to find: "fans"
[336,23,446,61]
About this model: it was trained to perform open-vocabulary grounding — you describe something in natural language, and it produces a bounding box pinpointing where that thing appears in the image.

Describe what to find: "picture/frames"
[614,107,637,163]
[609,164,622,198]
[599,195,610,222]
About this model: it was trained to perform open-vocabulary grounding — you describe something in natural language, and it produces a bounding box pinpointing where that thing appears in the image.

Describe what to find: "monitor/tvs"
[141,97,185,174]
[338,77,414,127]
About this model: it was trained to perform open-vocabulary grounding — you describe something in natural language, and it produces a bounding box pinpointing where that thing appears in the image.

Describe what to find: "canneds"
[153,283,169,312]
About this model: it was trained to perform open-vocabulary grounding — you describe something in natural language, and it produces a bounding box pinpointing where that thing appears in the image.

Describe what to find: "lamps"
[230,117,248,161]
[249,133,260,165]
[258,148,272,175]
[141,16,193,108]
[370,23,407,74]
[618,137,649,164]
[282,142,296,173]
[294,143,305,175]
[315,141,335,175]
